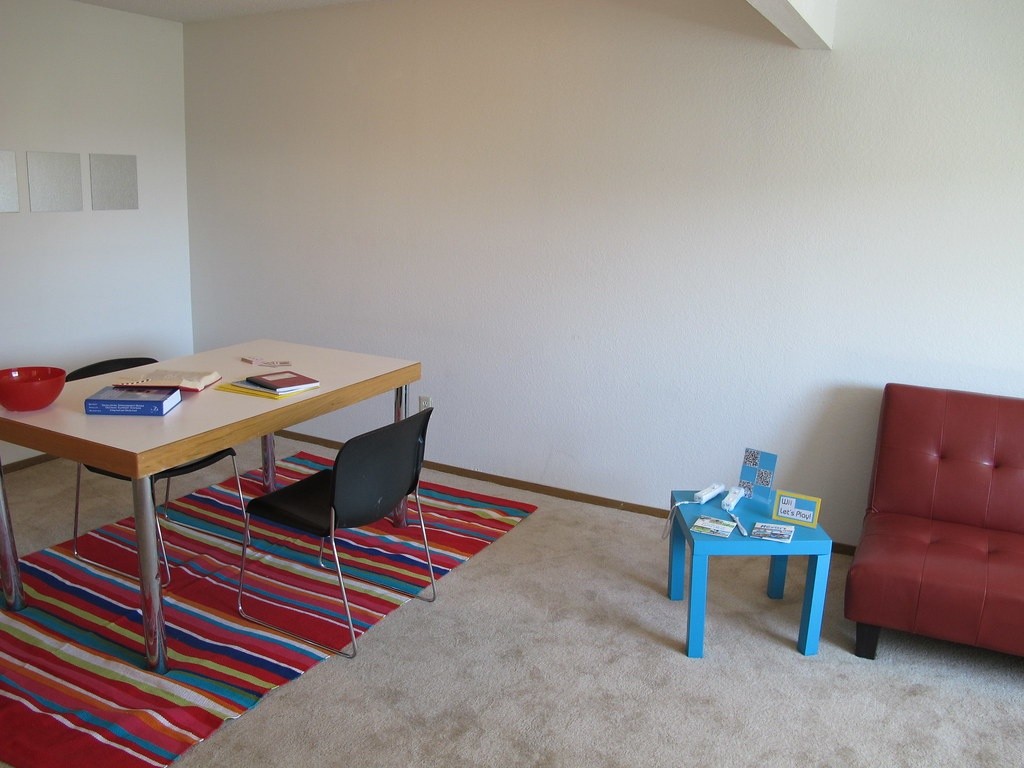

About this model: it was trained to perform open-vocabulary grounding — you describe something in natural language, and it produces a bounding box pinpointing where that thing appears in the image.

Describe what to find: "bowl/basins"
[0,366,67,412]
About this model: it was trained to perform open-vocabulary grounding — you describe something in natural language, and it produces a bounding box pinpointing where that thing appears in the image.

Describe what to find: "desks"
[0,338,422,672]
[668,488,835,659]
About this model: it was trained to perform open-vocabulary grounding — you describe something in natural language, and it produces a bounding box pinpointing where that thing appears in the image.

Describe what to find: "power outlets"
[418,395,432,413]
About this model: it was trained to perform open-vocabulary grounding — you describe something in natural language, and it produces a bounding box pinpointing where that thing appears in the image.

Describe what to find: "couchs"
[844,382,1024,660]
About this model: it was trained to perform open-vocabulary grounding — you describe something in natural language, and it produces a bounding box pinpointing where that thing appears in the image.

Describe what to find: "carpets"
[1,449,540,767]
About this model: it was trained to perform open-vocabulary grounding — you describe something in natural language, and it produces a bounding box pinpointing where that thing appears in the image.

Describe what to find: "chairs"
[237,407,439,658]
[61,356,252,584]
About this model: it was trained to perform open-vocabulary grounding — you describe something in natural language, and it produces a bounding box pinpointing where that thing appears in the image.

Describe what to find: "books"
[212,379,319,400]
[691,515,738,538]
[749,522,794,544]
[245,370,320,391]
[112,369,222,394]
[84,386,183,418]
